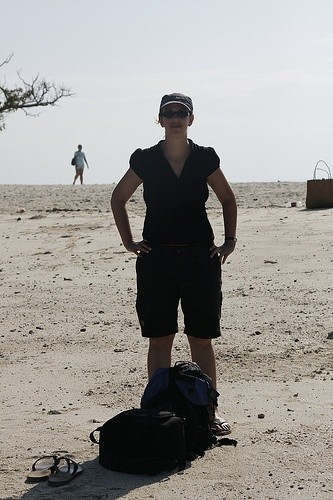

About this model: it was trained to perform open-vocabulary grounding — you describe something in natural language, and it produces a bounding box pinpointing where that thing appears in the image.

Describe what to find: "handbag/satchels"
[90,407,185,475]
[306,160,333,209]
[71,158,75,165]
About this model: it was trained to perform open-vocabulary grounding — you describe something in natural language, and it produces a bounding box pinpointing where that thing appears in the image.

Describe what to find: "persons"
[72,145,90,185]
[111,93,237,436]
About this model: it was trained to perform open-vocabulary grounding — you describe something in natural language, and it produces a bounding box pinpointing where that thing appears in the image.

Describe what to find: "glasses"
[161,110,190,118]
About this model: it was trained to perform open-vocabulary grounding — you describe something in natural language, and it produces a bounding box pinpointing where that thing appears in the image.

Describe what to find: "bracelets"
[225,236,237,242]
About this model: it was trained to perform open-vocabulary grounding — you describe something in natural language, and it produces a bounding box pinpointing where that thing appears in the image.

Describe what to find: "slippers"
[48,457,83,486]
[213,420,231,435]
[27,455,58,481]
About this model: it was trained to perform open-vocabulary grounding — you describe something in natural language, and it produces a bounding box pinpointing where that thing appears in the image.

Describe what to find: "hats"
[160,93,193,113]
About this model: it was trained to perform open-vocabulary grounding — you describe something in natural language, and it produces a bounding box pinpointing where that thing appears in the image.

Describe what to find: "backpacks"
[141,360,213,451]
